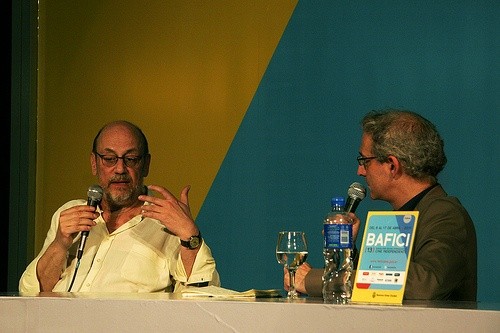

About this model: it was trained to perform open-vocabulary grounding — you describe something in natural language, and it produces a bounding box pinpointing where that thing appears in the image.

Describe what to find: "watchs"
[180,231,202,250]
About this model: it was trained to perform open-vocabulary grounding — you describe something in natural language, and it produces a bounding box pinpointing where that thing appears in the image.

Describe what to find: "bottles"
[321,197,353,301]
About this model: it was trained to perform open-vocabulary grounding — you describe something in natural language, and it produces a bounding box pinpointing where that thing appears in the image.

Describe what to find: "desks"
[0,295,499,333]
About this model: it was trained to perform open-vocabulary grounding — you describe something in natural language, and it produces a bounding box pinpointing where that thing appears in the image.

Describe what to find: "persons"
[18,119,219,297]
[284,107,478,309]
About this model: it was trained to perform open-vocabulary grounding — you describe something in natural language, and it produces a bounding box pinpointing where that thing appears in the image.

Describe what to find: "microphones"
[343,182,366,213]
[77,185,103,259]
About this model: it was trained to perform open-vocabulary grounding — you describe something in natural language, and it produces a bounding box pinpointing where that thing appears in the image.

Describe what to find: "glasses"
[94,150,144,168]
[357,154,384,165]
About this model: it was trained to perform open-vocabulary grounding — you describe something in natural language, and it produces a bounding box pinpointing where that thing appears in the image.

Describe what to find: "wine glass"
[276,231,308,301]
